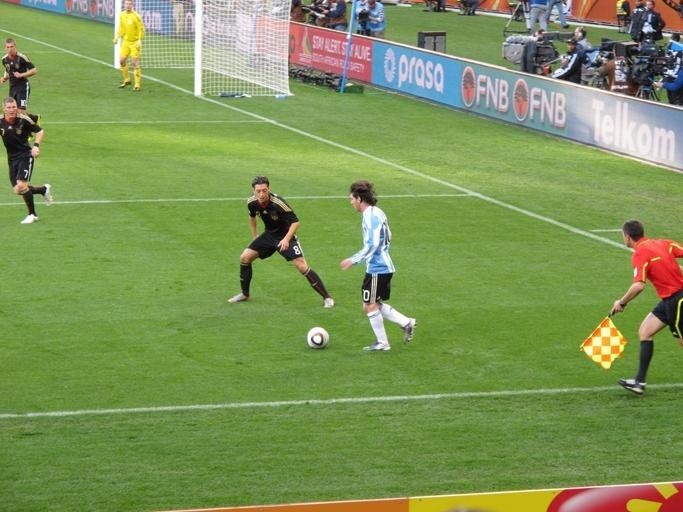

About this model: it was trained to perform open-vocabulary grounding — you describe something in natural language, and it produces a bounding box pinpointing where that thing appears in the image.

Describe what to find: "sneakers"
[616,377,647,394]
[228,294,249,303]
[36,115,41,124]
[42,184,52,207]
[401,318,416,345]
[118,81,132,89]
[20,214,38,224]
[324,298,334,310]
[27,134,35,143]
[363,343,391,351]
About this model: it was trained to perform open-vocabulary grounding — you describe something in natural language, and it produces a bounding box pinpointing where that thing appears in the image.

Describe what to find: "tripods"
[503,1,532,32]
[634,85,660,102]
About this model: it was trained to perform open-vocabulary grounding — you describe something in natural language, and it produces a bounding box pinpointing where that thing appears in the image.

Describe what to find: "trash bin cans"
[417,31,446,54]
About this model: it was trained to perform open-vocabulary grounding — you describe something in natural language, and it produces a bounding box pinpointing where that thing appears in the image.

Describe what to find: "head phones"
[577,29,584,39]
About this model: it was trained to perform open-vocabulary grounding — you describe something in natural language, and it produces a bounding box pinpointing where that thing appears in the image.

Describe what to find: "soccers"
[307,327,330,348]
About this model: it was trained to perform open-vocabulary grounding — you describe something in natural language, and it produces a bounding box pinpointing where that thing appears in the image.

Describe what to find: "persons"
[226,175,334,309]
[0,98,53,224]
[521,0,683,108]
[433,0,447,12]
[609,220,683,396]
[112,1,146,92]
[291,0,386,41]
[340,180,416,352]
[458,0,478,15]
[0,38,42,142]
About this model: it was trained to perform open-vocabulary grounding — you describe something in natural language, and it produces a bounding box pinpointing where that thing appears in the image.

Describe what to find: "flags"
[579,316,629,371]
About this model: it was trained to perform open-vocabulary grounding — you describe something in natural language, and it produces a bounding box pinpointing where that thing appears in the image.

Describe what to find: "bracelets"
[33,142,39,147]
